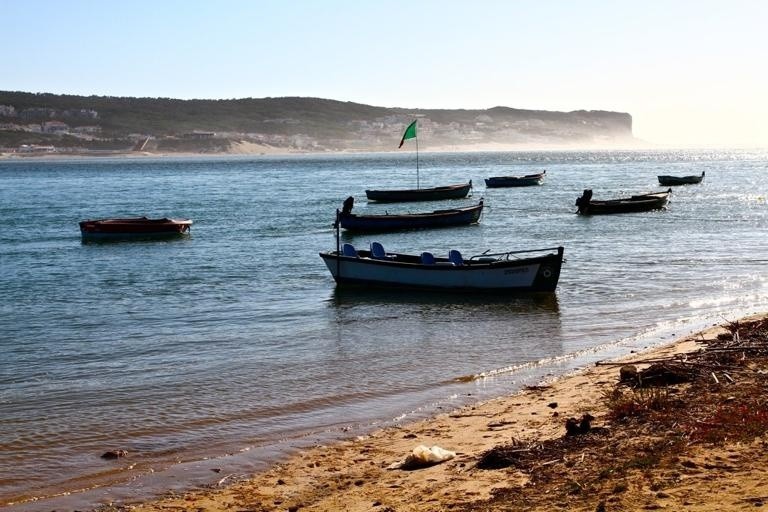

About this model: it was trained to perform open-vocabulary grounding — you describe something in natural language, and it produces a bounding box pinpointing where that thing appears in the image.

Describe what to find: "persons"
[330,196,354,229]
[574,189,592,214]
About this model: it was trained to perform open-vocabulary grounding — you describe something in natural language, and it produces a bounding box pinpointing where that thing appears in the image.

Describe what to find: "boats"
[657,171,706,186]
[365,179,473,201]
[484,169,547,187]
[318,247,565,292]
[573,188,672,213]
[79,216,192,236]
[331,196,484,230]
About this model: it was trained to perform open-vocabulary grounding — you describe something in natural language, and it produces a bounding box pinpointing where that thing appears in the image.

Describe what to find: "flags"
[398,120,415,148]
